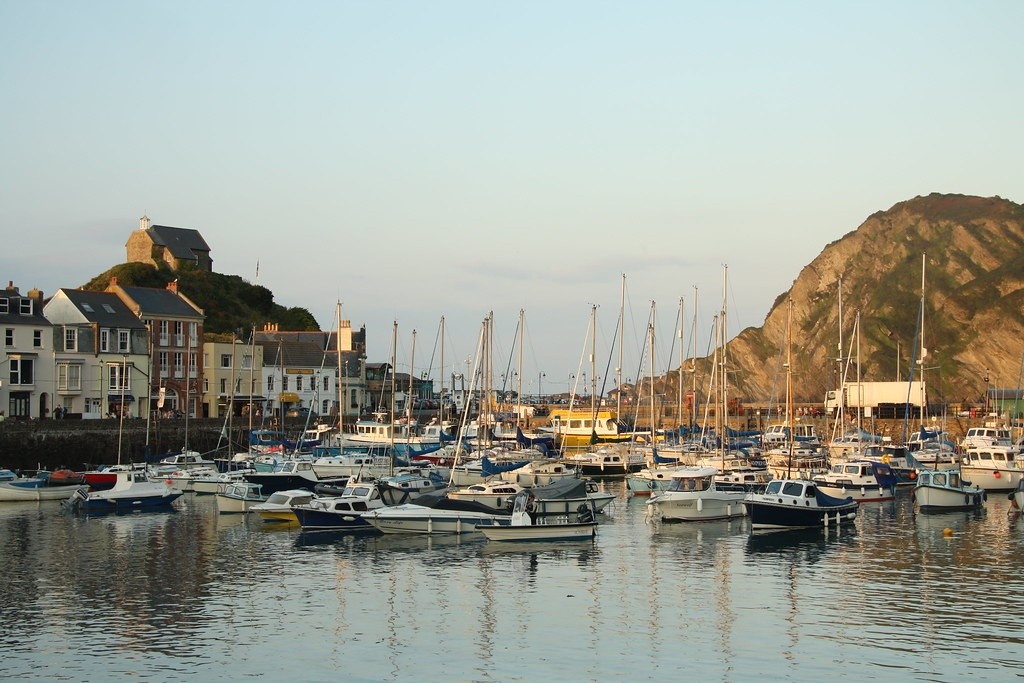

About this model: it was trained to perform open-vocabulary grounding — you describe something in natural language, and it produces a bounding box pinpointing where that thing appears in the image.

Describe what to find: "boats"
[0,251,1024,542]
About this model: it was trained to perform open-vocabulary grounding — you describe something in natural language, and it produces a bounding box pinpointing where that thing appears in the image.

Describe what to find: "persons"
[775,404,848,419]
[53,406,61,418]
[58,405,63,416]
[105,409,185,420]
[0,414,5,421]
[63,407,68,418]
